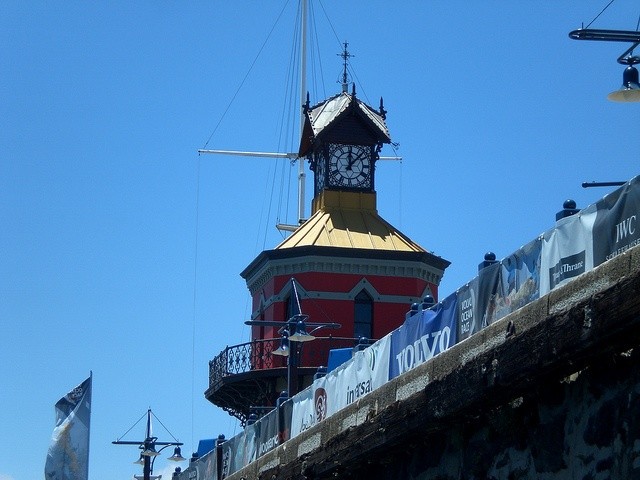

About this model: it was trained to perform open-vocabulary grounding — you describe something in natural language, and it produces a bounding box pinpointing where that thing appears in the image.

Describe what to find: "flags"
[45,377,92,480]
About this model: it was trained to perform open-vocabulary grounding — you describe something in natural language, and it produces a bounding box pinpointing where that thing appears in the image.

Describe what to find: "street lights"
[244,280,342,396]
[568,0,640,102]
[113,408,186,480]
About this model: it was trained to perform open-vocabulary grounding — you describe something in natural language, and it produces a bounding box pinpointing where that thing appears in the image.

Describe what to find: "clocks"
[315,149,326,191]
[328,144,371,187]
